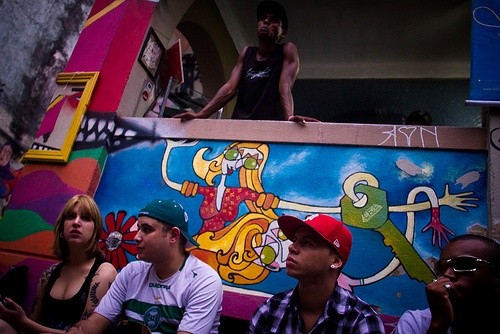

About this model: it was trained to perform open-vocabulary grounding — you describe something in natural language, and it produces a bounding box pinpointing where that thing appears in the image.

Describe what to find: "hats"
[278,214,351,265]
[138,199,201,247]
[258,3,288,33]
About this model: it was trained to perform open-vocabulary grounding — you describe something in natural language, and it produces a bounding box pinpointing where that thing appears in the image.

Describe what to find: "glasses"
[434,255,498,276]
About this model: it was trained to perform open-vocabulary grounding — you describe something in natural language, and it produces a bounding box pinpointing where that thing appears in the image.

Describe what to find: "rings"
[432,278,437,282]
[444,284,452,290]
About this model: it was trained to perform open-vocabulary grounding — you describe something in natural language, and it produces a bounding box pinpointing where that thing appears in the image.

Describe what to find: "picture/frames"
[24,71,100,164]
[137,26,166,83]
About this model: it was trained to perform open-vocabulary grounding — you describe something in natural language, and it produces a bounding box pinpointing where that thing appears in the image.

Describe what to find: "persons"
[171,0,320,126]
[391,232,500,333]
[247,212,385,334]
[64,197,223,333]
[1,192,118,334]
